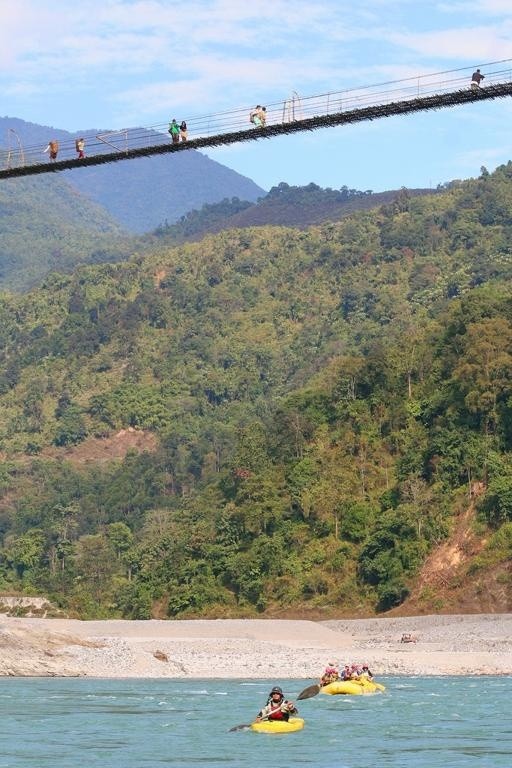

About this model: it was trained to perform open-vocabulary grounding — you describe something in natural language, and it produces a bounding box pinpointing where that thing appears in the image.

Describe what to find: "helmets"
[269,687,284,698]
[325,668,337,675]
[346,661,368,669]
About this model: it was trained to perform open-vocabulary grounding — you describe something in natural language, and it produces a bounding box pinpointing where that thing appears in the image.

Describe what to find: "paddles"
[228,684,321,734]
[370,681,386,692]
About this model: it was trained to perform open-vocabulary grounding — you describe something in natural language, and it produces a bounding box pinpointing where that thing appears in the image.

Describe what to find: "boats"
[320,679,384,695]
[249,719,304,734]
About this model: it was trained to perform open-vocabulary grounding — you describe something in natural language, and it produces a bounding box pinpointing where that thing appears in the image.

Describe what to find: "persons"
[321,661,374,687]
[471,69,485,90]
[171,119,180,143]
[77,139,84,158]
[252,105,263,129]
[256,687,299,723]
[261,107,267,127]
[180,121,187,141]
[43,140,58,163]
